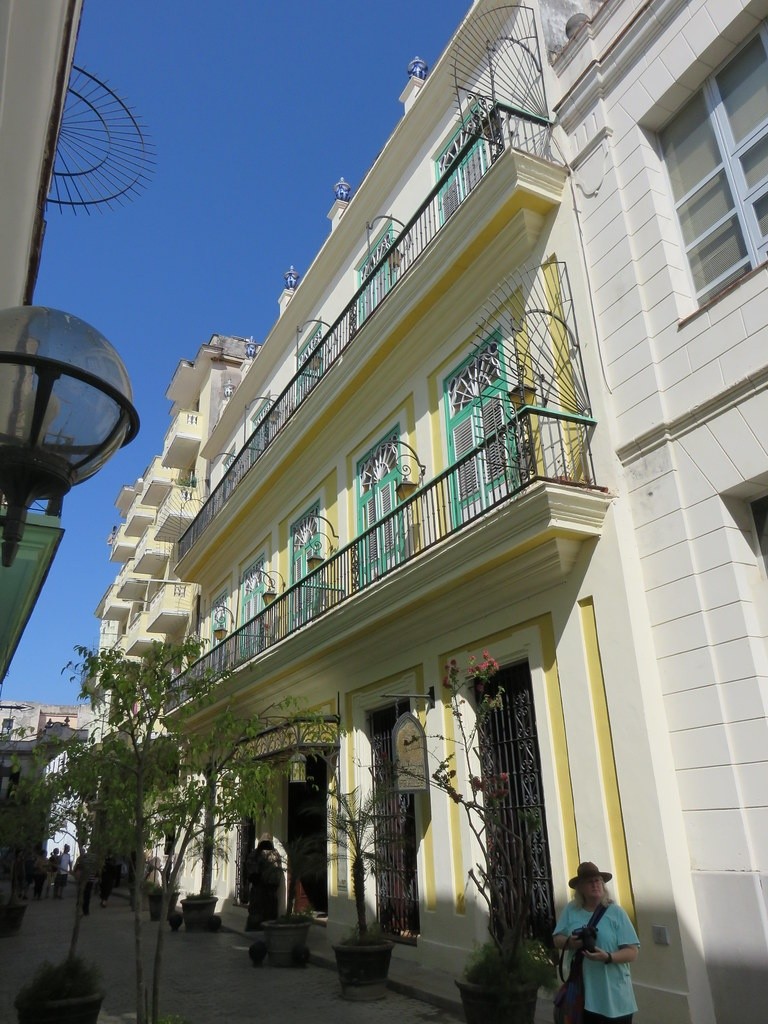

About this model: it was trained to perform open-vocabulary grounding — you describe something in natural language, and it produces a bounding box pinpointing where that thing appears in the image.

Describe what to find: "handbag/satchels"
[552,972,587,1024]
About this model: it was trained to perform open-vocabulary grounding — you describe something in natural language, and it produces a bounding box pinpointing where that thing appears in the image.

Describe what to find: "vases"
[453,976,541,1024]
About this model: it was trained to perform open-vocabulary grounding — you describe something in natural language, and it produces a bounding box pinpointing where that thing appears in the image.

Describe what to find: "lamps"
[466,93,507,145]
[296,320,338,371]
[366,215,413,271]
[245,395,288,425]
[203,606,235,642]
[371,441,427,502]
[505,308,581,417]
[211,452,243,484]
[295,516,338,573]
[240,569,286,609]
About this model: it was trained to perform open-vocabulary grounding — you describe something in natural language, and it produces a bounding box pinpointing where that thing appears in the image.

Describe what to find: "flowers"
[378,651,558,995]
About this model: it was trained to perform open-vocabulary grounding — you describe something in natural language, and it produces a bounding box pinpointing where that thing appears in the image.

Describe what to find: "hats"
[569,862,614,891]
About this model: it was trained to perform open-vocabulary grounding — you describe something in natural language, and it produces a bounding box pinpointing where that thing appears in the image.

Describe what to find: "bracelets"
[605,952,612,964]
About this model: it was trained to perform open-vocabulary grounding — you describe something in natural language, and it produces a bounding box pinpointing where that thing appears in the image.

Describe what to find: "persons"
[73,849,87,870]
[32,849,51,901]
[113,853,123,887]
[10,848,35,900]
[51,844,72,900]
[100,848,118,907]
[551,862,640,1024]
[83,870,96,916]
[45,848,61,898]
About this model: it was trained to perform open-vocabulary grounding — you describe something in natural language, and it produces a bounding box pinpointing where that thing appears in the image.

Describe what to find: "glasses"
[581,879,603,885]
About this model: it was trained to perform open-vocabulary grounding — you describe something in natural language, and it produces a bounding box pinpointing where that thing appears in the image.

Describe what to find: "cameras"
[572,924,597,952]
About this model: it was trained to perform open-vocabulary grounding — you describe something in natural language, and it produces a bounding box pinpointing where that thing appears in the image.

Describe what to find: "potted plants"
[0,636,418,1023]
